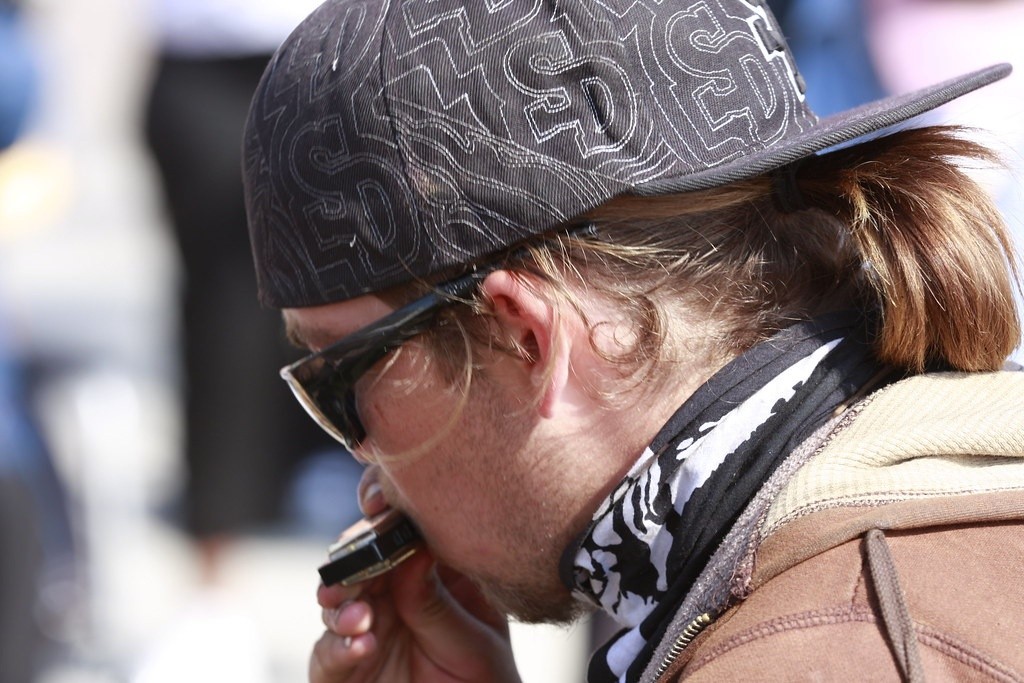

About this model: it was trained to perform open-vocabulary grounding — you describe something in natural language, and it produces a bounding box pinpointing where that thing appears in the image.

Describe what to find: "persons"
[239,0,1024,683]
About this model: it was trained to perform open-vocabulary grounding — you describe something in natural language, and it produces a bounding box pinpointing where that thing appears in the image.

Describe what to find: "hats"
[240,0,1014,311]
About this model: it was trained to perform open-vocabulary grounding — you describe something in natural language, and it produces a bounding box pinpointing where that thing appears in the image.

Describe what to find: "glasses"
[280,222,602,450]
